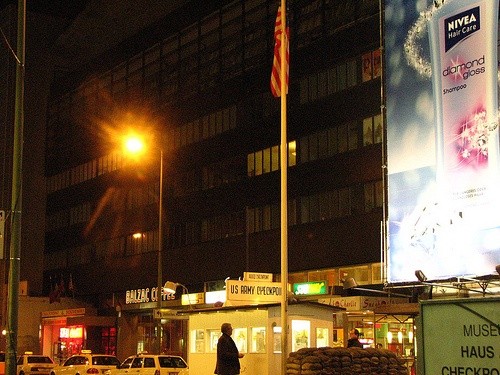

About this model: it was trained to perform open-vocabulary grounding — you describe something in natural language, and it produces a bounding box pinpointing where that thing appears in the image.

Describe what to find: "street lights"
[121,131,163,364]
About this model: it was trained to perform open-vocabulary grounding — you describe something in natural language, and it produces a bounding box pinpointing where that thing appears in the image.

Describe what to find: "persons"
[214,323,244,375]
[347,328,364,349]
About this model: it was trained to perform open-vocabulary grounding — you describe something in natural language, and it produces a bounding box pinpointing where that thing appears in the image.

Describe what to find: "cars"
[17,351,56,375]
[106,351,189,375]
[49,350,121,375]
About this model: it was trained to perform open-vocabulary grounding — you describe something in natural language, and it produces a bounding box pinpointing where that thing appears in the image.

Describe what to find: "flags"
[270,0,290,97]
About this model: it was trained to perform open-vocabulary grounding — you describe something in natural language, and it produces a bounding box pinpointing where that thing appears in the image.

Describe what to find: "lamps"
[341,277,358,289]
[416,271,426,282]
[162,281,196,310]
